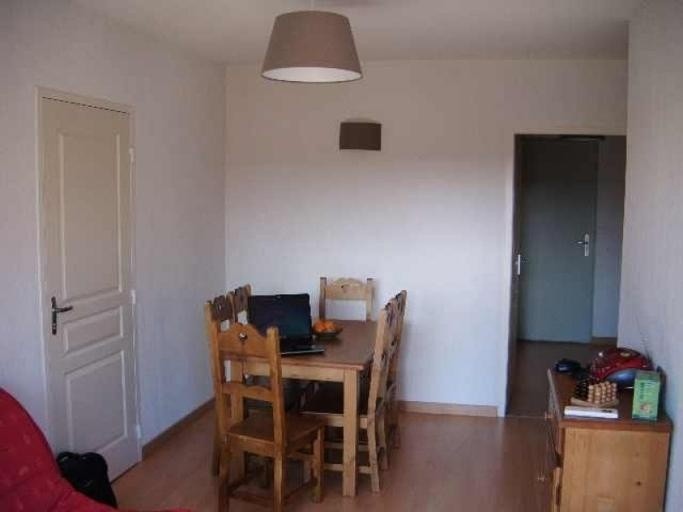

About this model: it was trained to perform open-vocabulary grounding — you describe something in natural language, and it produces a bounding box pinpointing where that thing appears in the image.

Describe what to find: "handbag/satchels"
[55,451,116,507]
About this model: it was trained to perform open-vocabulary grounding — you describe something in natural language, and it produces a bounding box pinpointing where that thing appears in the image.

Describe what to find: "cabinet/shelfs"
[535,366,672,511]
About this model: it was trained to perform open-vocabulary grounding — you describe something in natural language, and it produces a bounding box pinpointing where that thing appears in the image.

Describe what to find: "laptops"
[247,294,325,356]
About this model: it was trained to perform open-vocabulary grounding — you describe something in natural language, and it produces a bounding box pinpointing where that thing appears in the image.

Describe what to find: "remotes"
[564,405,619,419]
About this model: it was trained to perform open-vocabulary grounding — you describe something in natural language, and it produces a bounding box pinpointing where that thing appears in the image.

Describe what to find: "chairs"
[209,321,328,511]
[0,387,117,511]
[318,277,374,320]
[306,289,407,503]
[203,282,316,478]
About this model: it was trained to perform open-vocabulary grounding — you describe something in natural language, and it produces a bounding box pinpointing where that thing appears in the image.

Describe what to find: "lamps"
[257,2,364,85]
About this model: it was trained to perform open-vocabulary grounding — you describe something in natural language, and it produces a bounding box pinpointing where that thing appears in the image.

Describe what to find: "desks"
[229,318,379,497]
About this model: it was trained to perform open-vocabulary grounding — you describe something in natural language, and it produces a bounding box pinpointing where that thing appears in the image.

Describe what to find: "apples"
[313,320,335,333]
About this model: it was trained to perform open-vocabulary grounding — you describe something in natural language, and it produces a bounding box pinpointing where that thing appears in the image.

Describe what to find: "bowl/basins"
[311,325,343,340]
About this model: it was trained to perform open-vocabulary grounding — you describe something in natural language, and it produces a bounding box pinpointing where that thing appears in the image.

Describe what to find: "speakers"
[340,123,381,151]
